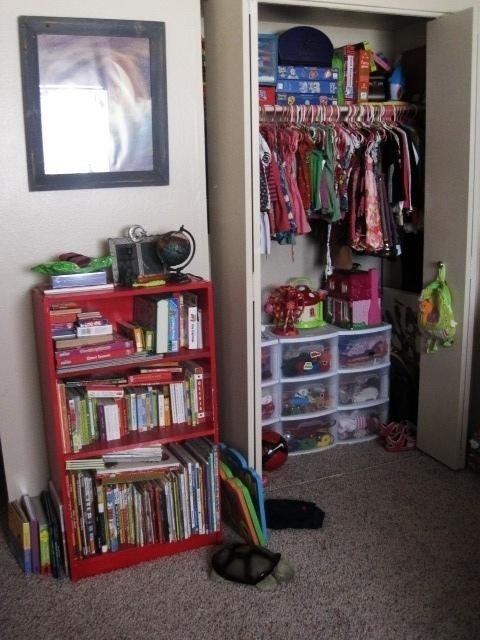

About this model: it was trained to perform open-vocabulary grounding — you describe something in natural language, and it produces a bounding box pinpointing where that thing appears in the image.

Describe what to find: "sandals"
[378,420,418,441]
[386,431,417,452]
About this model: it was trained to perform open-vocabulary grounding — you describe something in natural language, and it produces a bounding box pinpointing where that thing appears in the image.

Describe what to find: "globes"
[156,225,196,284]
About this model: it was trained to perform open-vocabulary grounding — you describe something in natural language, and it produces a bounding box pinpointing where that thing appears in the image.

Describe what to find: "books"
[50,291,204,374]
[331,39,391,105]
[5,481,67,579]
[56,359,219,559]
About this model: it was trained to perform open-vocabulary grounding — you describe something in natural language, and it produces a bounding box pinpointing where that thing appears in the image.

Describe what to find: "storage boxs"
[259,35,278,87]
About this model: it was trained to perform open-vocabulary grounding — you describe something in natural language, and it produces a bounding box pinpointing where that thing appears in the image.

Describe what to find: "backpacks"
[415,264,457,355]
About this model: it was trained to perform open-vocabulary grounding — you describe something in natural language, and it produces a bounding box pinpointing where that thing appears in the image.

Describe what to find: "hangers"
[258,99,417,137]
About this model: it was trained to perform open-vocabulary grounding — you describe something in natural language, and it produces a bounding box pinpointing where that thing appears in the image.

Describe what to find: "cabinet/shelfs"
[260,322,390,456]
[32,274,225,580]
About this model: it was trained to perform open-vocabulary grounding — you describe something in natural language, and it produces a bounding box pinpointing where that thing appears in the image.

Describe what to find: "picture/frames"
[17,15,168,191]
[108,237,138,285]
[136,235,172,281]
